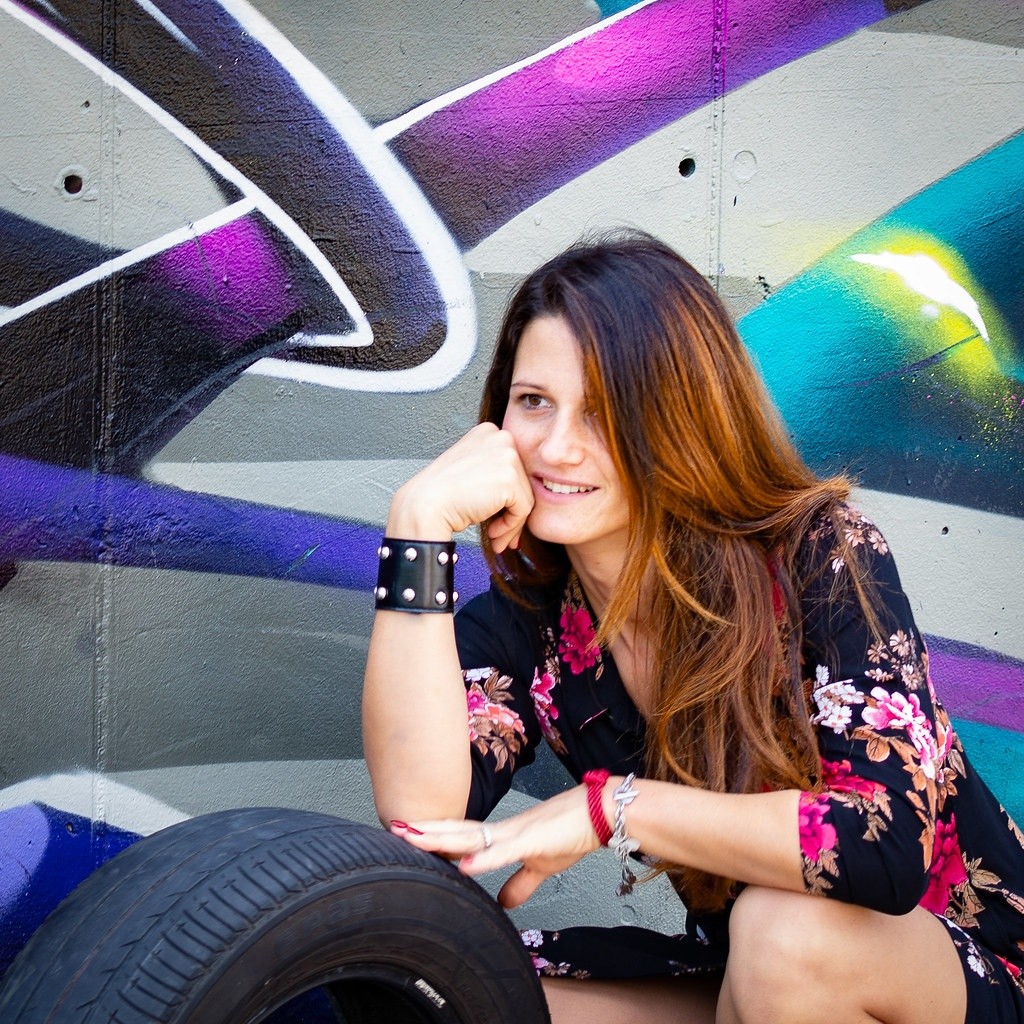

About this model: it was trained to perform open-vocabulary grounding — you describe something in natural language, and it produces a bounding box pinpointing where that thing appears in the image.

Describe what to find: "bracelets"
[583,768,640,896]
[372,537,458,613]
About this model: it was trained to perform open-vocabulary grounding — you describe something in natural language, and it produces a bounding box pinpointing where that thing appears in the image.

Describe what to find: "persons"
[359,236,1024,1023]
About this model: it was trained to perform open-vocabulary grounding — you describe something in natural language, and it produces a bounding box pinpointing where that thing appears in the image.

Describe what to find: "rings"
[480,822,492,849]
